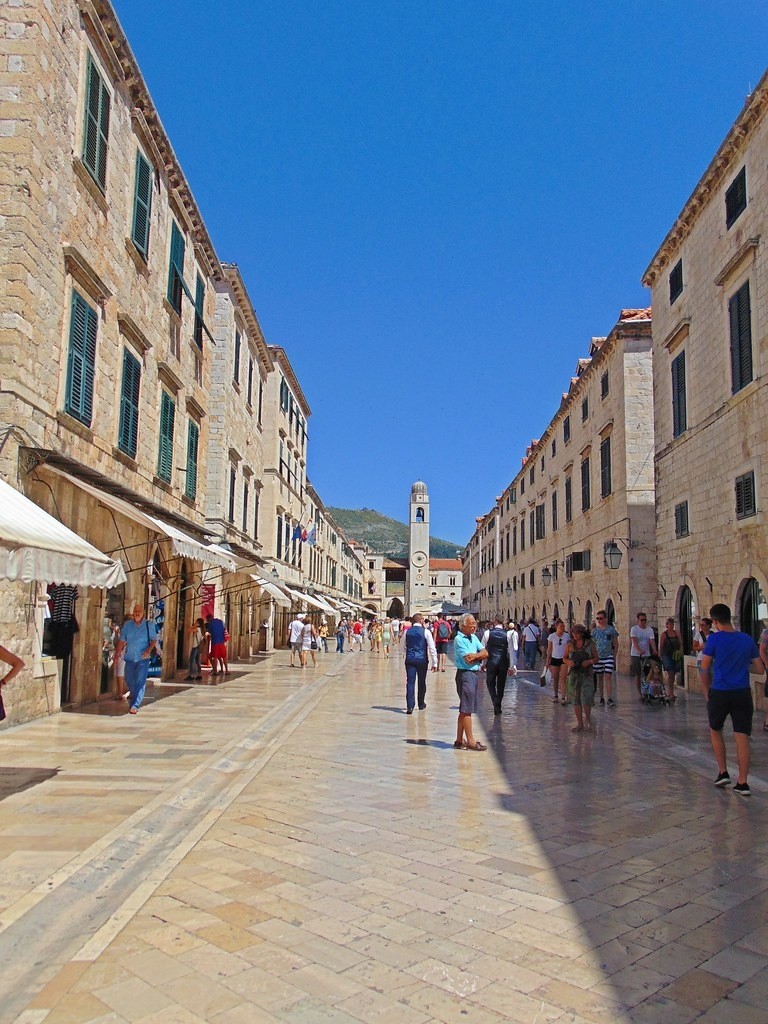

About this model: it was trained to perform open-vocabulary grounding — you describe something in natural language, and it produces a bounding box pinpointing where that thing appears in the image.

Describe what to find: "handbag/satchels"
[539,666,551,687]
[287,638,292,649]
[224,626,231,642]
[150,646,157,658]
[311,641,317,649]
[673,648,684,662]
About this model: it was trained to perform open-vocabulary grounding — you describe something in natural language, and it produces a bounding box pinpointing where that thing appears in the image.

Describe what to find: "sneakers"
[732,782,751,795]
[715,770,731,785]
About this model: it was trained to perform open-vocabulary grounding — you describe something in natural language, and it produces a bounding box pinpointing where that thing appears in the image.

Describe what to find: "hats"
[570,624,585,632]
[297,614,304,618]
[495,613,505,622]
[508,622,514,629]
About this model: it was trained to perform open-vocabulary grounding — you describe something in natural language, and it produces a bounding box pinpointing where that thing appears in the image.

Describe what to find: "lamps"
[505,578,519,596]
[487,592,499,603]
[540,560,564,586]
[603,535,631,569]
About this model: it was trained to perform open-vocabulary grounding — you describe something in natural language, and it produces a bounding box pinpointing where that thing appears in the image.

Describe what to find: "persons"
[317,621,329,653]
[659,618,683,700]
[335,615,598,707]
[758,628,768,732]
[693,618,715,694]
[288,614,307,667]
[205,623,223,674]
[647,664,667,698]
[184,617,204,680]
[0,646,25,721]
[591,610,620,707]
[402,613,438,715]
[564,624,599,732]
[302,617,318,670]
[205,614,231,675]
[453,613,488,751]
[701,603,764,795]
[113,605,156,715]
[113,614,135,700]
[630,612,658,701]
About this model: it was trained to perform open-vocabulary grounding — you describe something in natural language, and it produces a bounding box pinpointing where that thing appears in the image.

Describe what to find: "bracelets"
[2,679,6,685]
[590,660,593,664]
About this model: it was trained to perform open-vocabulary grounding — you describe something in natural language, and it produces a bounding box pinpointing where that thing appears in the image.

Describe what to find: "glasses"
[559,638,562,645]
[596,617,605,620]
[572,631,579,634]
[699,623,706,627]
[639,618,647,622]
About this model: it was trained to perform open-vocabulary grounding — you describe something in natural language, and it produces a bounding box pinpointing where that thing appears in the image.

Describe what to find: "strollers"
[639,651,671,706]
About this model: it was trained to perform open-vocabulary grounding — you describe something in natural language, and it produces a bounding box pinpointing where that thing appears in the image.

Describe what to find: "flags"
[291,523,316,546]
[371,586,376,592]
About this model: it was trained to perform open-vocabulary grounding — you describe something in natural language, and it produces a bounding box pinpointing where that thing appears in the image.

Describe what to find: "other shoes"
[291,659,320,668]
[184,668,232,681]
[114,690,137,714]
[410,693,677,751]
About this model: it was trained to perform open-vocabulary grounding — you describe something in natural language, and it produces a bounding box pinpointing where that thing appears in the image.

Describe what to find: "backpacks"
[436,621,448,638]
[196,626,207,653]
[568,640,593,671]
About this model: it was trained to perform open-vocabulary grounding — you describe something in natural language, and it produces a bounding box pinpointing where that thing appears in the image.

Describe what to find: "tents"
[419,602,478,620]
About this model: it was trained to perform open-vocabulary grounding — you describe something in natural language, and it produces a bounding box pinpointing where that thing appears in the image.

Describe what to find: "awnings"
[0,478,127,589]
[211,544,375,616]
[40,464,237,574]
